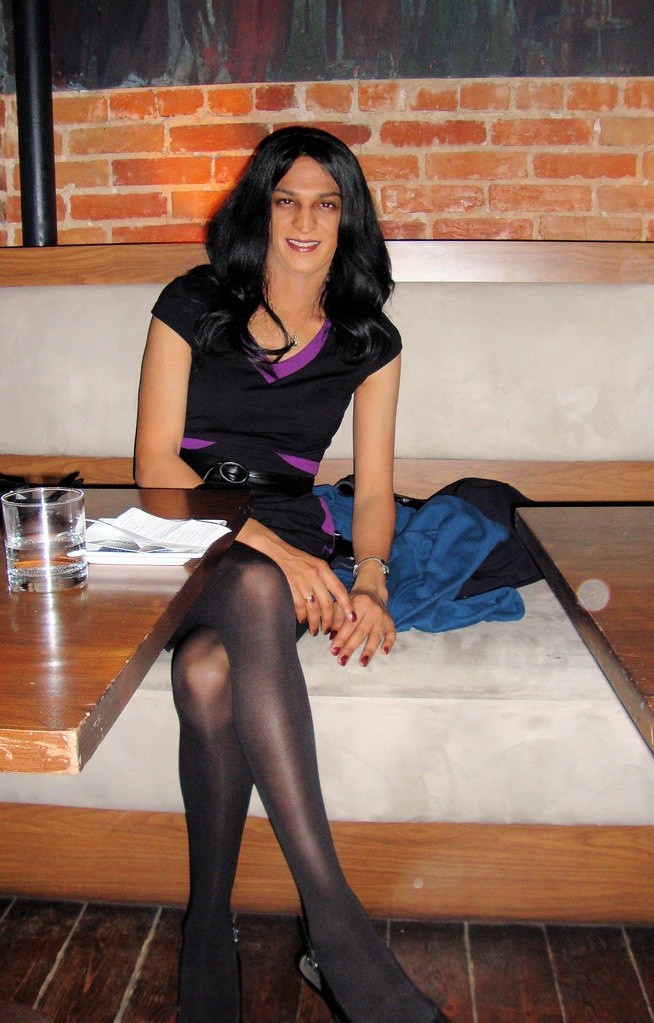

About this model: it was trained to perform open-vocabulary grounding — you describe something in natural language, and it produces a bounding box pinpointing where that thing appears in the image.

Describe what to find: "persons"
[135,126,450,1023]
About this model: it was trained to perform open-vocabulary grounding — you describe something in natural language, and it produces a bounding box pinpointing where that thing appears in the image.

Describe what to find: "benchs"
[1,444,654,929]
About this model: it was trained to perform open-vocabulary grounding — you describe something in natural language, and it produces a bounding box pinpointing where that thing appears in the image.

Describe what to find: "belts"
[179,450,315,494]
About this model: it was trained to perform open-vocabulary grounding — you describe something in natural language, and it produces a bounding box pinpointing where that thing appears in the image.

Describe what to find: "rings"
[304,595,317,603]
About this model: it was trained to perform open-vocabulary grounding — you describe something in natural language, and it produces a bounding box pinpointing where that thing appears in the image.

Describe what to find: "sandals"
[294,911,453,1023]
[177,907,244,1023]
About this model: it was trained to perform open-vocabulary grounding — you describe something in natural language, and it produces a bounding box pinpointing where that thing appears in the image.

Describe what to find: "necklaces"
[280,326,300,349]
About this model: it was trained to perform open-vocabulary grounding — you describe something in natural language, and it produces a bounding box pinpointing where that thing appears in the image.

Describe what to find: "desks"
[1,484,255,778]
[508,501,654,756]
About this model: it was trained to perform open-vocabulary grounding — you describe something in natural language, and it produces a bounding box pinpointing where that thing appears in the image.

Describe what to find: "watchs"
[353,557,390,580]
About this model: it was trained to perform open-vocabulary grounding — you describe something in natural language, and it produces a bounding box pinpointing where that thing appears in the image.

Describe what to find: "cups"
[1,487,89,595]
